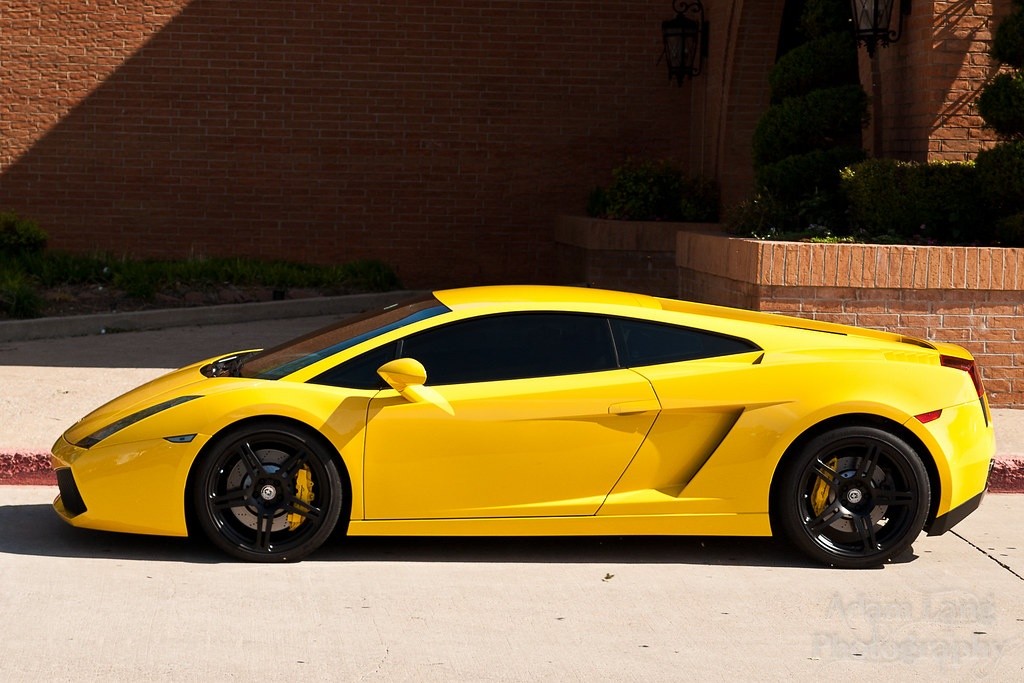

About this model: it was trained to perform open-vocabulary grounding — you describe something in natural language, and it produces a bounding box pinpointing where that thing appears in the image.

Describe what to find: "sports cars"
[49,283,996,569]
